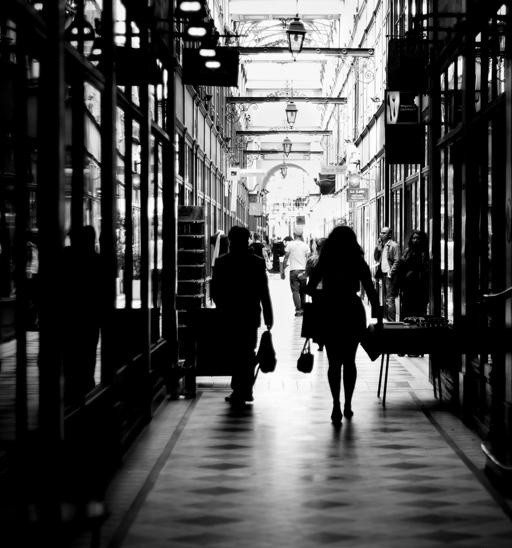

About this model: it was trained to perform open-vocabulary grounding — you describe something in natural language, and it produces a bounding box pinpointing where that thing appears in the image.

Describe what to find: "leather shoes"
[232,405,251,414]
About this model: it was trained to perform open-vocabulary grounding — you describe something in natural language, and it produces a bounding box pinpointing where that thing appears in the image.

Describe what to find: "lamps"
[283,131,293,157]
[279,153,287,178]
[285,0,308,60]
[284,86,298,129]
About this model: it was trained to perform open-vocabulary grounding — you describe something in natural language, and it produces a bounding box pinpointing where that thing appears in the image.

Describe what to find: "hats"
[294,228,303,235]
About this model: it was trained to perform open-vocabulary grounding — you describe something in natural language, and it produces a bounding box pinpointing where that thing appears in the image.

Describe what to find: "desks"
[186,304,237,377]
[373,318,446,410]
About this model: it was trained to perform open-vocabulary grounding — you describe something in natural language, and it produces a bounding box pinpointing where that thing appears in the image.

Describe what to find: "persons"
[302,225,381,430]
[251,230,324,317]
[211,225,273,413]
[372,226,401,321]
[384,229,429,361]
[51,225,103,404]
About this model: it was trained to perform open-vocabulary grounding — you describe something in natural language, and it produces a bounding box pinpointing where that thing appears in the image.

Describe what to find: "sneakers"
[294,311,300,316]
[300,309,303,315]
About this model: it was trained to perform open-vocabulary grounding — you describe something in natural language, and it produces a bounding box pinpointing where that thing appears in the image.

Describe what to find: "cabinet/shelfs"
[175,202,210,342]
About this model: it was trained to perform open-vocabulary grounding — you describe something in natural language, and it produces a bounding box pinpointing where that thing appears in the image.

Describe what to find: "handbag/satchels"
[254,327,275,377]
[298,338,313,371]
